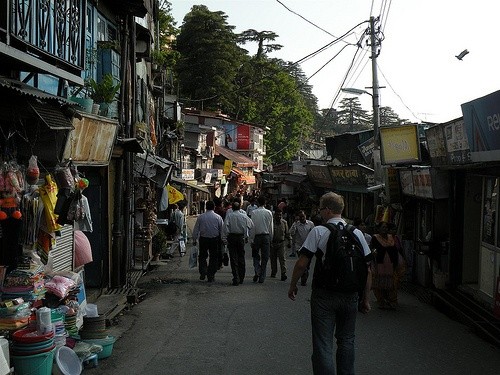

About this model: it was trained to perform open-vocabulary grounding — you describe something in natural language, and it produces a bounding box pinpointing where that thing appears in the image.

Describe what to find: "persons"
[289,210,314,286]
[223,202,255,286]
[200,198,205,214]
[200,186,295,249]
[352,219,409,313]
[288,191,372,375]
[309,212,325,225]
[170,202,187,258]
[192,200,224,283]
[248,198,273,283]
[227,134,232,142]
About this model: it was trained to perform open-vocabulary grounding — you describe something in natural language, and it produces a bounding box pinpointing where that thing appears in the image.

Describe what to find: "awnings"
[174,176,211,197]
[0,74,86,129]
[216,146,258,167]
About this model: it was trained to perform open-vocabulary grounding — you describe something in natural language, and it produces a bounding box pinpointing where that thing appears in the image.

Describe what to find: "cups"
[36,308,51,325]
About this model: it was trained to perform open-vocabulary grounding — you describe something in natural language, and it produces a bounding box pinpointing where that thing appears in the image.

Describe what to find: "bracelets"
[193,240,196,242]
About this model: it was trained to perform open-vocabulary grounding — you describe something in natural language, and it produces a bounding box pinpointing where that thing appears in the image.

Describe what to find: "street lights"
[340,88,383,185]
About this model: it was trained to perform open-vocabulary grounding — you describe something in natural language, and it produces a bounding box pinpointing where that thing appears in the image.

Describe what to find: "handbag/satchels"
[188,245,199,268]
[358,268,378,313]
[164,212,179,236]
[222,245,229,266]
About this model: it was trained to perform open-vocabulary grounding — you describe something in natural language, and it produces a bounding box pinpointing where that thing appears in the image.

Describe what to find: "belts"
[228,233,244,236]
[255,234,269,236]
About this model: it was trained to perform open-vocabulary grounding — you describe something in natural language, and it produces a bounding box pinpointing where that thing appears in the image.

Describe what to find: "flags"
[149,100,157,146]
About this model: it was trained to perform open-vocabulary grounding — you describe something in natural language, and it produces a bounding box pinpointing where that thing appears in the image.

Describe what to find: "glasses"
[318,208,331,213]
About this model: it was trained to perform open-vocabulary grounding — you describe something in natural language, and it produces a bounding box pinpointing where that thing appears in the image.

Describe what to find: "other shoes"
[271,273,275,278]
[253,274,264,283]
[281,276,287,281]
[232,282,243,285]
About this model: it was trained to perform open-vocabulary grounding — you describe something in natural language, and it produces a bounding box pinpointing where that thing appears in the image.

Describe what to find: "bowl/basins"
[52,346,82,375]
[81,340,116,359]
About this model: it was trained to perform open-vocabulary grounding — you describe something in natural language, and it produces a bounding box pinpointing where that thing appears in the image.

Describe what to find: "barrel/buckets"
[10,328,56,375]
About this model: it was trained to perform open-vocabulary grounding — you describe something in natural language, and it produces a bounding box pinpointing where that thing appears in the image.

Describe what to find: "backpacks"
[316,221,367,298]
